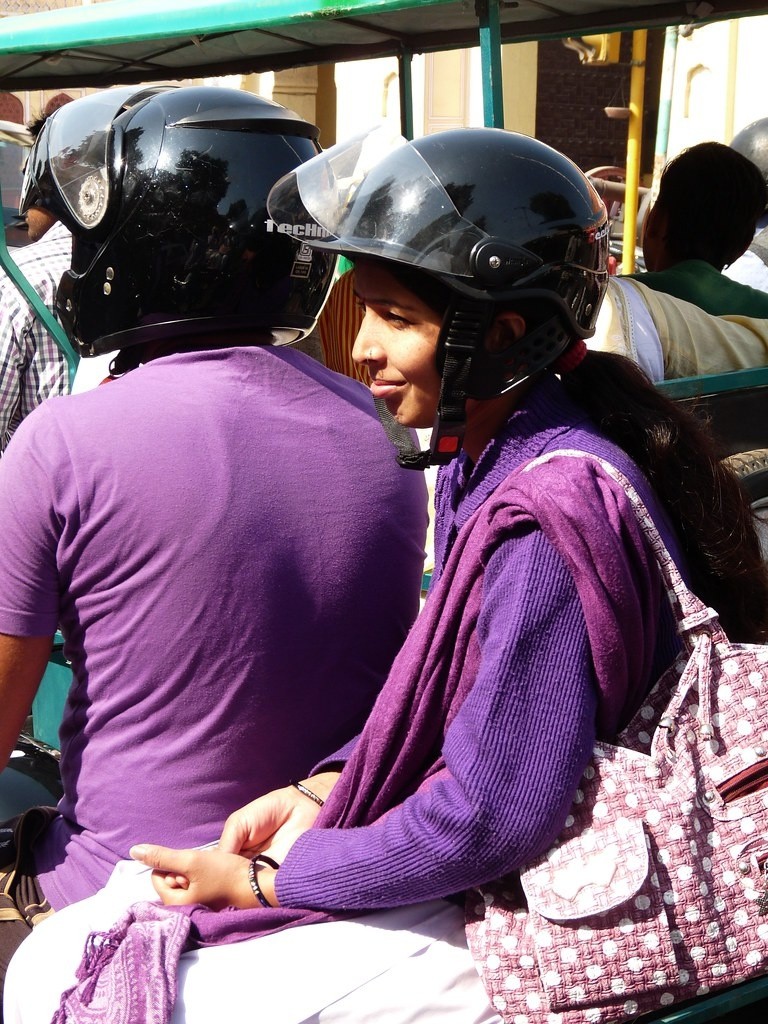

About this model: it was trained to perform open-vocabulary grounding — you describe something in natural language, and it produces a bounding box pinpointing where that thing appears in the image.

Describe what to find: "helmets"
[730,117,768,212]
[267,122,612,340]
[18,84,338,359]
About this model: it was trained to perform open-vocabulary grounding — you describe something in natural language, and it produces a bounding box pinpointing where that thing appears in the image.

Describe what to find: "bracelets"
[247,854,280,908]
[290,776,325,807]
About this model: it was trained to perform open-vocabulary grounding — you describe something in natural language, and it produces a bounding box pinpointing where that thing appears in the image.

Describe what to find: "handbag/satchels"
[460,450,768,1024]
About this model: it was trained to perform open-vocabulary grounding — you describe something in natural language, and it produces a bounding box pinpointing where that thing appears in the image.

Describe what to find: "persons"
[128,126,768,1024]
[0,86,430,1024]
